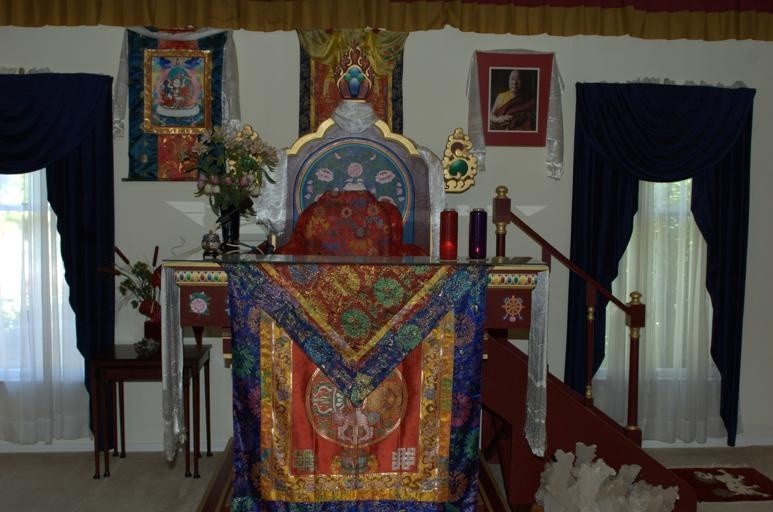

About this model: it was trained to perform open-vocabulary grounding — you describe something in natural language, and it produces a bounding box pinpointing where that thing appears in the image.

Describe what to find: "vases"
[214,217,247,260]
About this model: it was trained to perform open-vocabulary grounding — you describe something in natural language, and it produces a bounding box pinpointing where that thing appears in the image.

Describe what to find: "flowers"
[178,115,280,224]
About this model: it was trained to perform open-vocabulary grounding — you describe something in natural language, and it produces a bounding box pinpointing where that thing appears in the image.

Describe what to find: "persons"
[490,71,535,130]
[159,79,176,109]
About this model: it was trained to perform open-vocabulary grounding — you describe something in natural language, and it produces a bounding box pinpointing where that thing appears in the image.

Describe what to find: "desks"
[160,252,551,468]
[85,341,214,479]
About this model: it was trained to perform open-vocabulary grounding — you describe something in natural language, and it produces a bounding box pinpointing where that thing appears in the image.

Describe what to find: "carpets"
[667,465,773,503]
